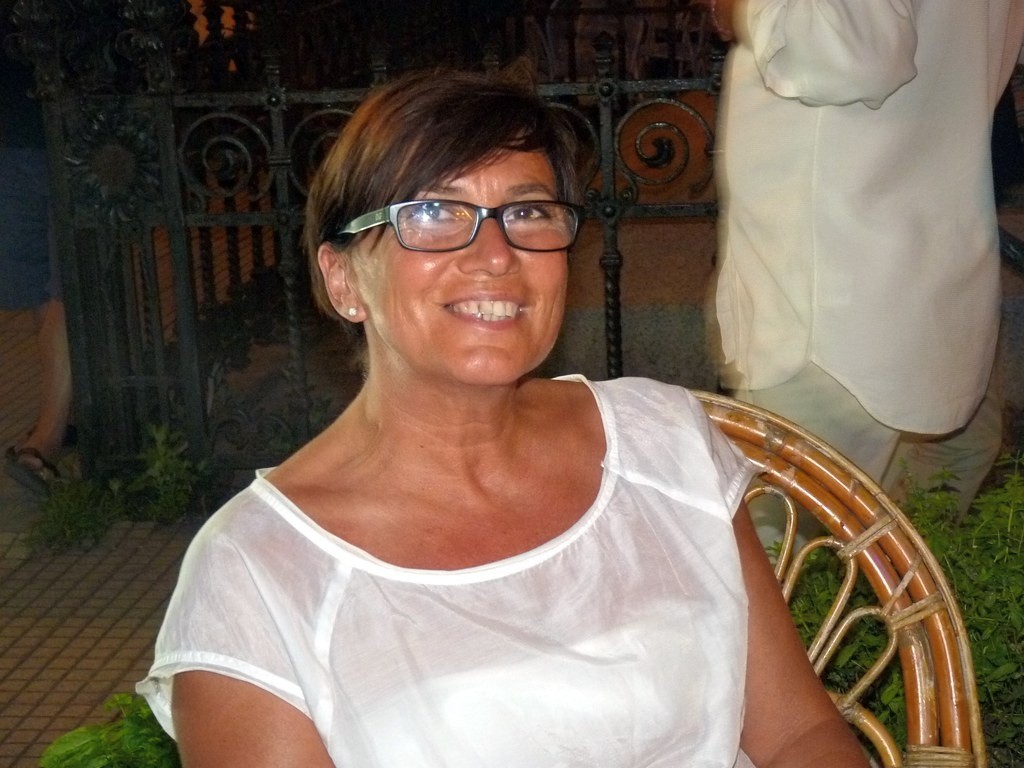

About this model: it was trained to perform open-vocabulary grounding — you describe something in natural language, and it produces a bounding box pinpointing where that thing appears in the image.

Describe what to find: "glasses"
[335,197,587,254]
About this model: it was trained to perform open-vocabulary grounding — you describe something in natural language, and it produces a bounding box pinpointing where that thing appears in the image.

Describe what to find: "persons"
[135,68,872,767]
[711,1,1024,570]
[0,1,78,497]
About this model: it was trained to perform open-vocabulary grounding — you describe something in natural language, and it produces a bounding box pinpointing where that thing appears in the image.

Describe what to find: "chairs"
[687,389,987,768]
